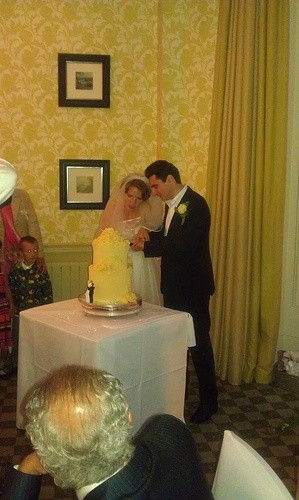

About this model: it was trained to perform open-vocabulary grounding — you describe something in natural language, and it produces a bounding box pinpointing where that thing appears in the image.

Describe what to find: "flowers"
[174,200,189,225]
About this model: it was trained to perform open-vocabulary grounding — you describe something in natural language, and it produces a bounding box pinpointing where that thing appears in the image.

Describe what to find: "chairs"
[211,430,296,500]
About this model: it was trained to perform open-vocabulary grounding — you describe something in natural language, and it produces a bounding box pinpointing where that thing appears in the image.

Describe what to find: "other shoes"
[190,406,218,424]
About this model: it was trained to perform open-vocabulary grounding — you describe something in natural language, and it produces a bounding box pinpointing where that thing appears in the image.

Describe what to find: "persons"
[92,173,164,307]
[0,236,53,379]
[128,160,219,424]
[0,365,215,500]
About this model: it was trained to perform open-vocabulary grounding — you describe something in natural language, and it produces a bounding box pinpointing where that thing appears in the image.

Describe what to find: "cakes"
[86,227,136,303]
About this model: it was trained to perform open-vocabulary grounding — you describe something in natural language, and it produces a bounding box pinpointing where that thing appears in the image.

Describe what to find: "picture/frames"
[57,53,111,107]
[59,158,110,210]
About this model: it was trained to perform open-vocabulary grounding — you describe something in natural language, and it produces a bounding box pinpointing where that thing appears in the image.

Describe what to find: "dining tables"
[15,296,196,437]
